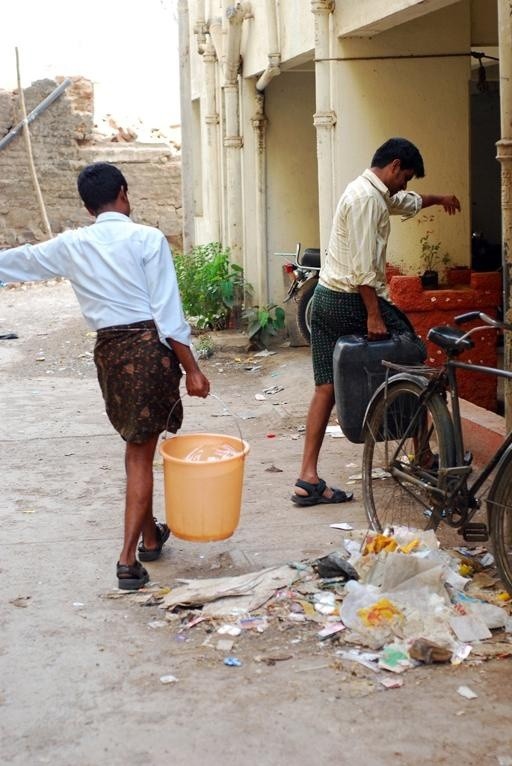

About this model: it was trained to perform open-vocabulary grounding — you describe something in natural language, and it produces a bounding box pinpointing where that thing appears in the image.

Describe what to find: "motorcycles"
[274,241,319,345]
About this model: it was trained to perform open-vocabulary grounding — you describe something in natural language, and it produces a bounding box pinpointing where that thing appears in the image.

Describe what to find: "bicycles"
[359,311,511,595]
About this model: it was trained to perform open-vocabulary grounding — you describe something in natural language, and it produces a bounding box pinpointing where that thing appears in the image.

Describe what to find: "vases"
[446,267,472,287]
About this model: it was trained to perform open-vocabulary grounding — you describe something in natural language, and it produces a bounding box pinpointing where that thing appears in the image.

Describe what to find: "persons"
[290,138,462,508]
[0,164,210,591]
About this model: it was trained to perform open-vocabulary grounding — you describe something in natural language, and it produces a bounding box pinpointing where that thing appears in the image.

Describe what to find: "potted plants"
[420,230,450,290]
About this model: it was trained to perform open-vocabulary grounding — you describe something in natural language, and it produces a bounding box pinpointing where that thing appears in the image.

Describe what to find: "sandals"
[412,450,472,475]
[116,560,149,589]
[291,478,353,506]
[138,516,170,561]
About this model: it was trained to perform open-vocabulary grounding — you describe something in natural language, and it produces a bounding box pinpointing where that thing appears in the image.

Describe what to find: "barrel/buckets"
[332,330,426,443]
[157,392,251,542]
